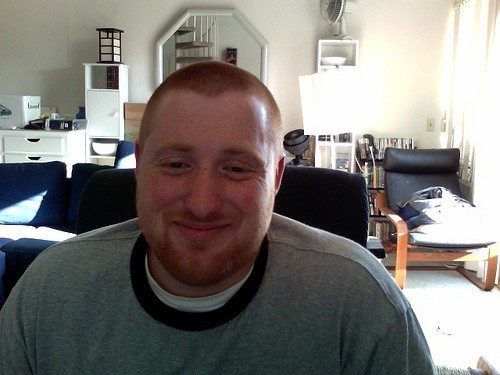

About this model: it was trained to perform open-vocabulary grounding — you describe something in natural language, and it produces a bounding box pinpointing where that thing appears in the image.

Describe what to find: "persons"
[0,61,438,375]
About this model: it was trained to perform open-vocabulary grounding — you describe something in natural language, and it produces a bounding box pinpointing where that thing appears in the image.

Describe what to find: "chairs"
[79,166,368,257]
[374,148,500,291]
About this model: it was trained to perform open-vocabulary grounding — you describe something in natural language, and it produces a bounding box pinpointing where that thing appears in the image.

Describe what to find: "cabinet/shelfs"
[315,39,359,172]
[355,154,413,262]
[0,129,86,178]
[83,62,130,167]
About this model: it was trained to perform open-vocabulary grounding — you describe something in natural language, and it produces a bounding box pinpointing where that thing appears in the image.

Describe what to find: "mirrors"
[155,7,268,87]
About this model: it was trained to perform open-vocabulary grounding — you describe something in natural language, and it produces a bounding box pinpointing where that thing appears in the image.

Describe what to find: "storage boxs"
[0,95,42,129]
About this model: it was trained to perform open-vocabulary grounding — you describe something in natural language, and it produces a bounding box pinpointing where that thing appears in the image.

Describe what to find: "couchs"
[0,161,113,314]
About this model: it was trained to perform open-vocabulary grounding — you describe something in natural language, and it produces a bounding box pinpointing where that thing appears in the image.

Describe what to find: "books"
[356,138,415,241]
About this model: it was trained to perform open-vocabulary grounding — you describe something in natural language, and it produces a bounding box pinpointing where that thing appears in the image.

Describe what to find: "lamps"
[95,27,124,63]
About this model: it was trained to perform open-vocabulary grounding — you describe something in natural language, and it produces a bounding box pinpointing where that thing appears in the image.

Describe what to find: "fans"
[320,0,354,39]
